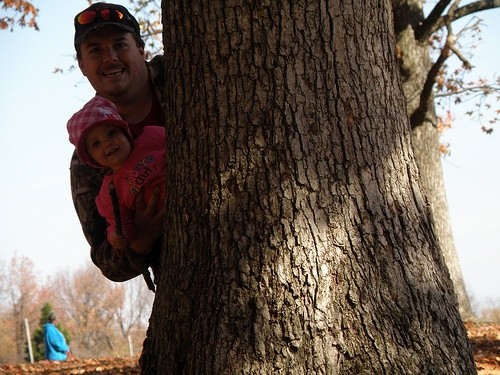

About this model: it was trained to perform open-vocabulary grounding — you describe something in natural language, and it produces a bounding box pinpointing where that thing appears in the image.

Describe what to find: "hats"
[66,96,133,169]
[73,2,141,51]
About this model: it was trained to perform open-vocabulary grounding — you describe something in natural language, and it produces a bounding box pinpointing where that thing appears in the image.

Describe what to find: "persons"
[70,2,164,285]
[43,312,70,360]
[67,97,167,249]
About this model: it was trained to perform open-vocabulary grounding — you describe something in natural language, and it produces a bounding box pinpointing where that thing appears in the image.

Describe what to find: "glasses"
[78,8,124,25]
